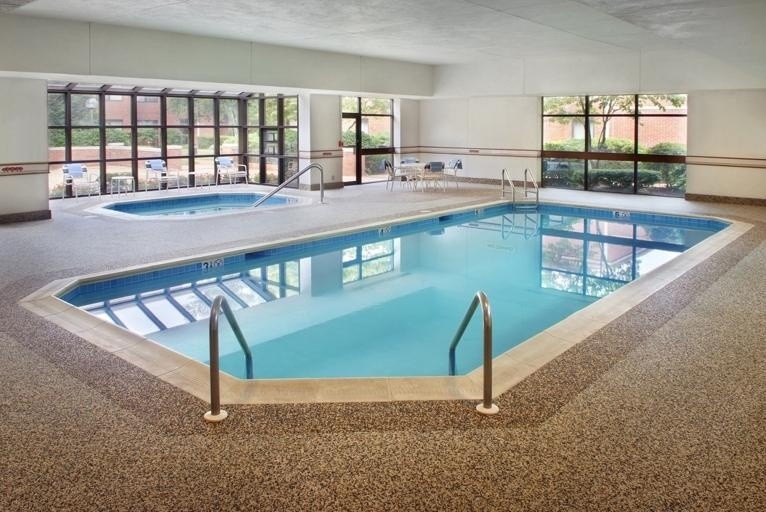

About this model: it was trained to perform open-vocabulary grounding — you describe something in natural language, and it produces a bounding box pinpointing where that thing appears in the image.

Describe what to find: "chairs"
[383,157,463,193]
[215,157,248,186]
[145,160,179,192]
[62,164,101,203]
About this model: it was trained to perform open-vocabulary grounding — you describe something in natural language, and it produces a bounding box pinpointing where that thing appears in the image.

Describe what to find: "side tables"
[187,172,210,190]
[111,175,135,198]
